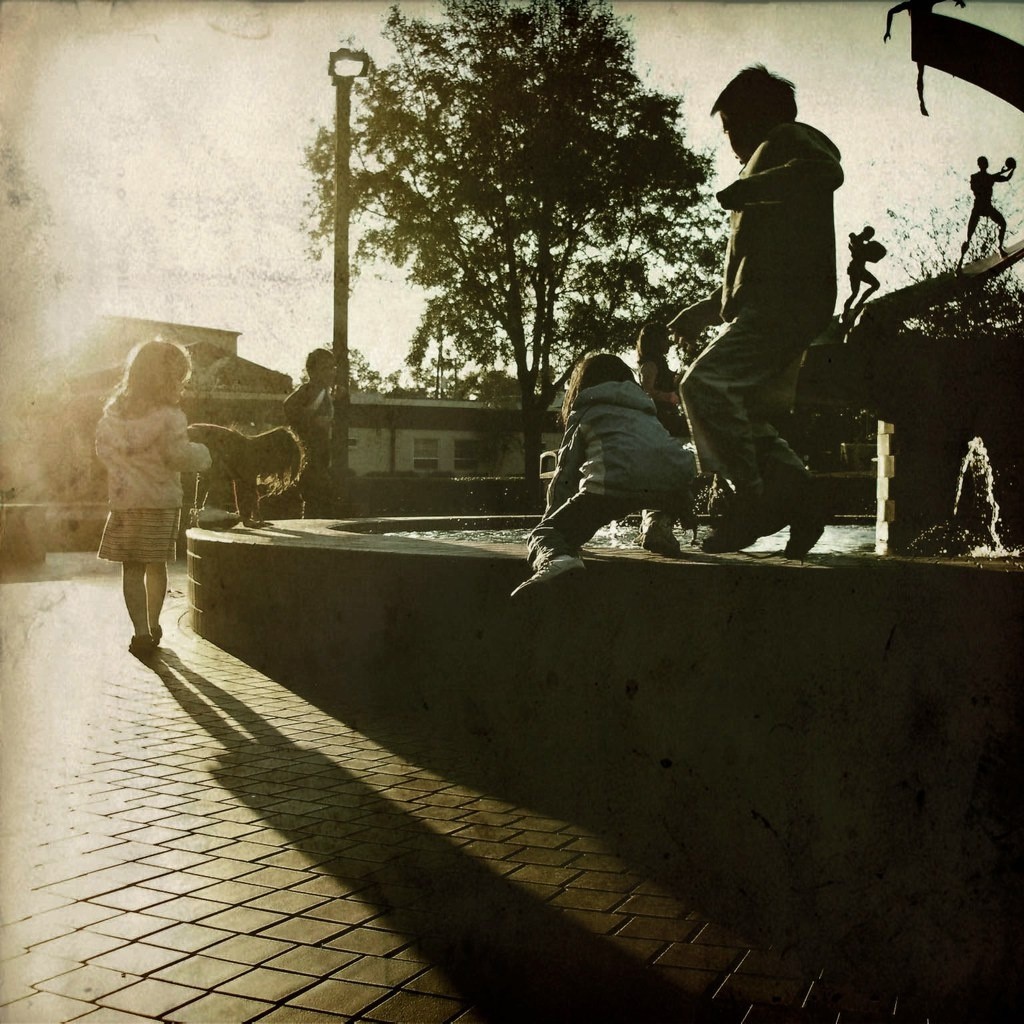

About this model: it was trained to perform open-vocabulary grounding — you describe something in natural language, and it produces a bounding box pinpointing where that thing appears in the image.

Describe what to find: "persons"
[512,353,698,608]
[93,335,215,658]
[282,348,348,520]
[173,422,308,531]
[839,226,887,324]
[637,323,693,543]
[884,0,968,118]
[954,156,1016,278]
[666,62,846,559]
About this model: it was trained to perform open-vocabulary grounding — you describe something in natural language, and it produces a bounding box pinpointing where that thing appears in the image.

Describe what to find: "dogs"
[186,422,307,528]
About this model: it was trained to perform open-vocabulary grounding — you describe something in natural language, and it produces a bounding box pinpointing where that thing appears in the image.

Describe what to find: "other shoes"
[129,626,162,652]
[302,504,328,519]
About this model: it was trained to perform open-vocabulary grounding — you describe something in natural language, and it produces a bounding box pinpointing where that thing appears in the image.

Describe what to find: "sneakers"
[699,487,833,559]
[189,507,241,530]
[642,520,680,558]
[511,554,586,602]
